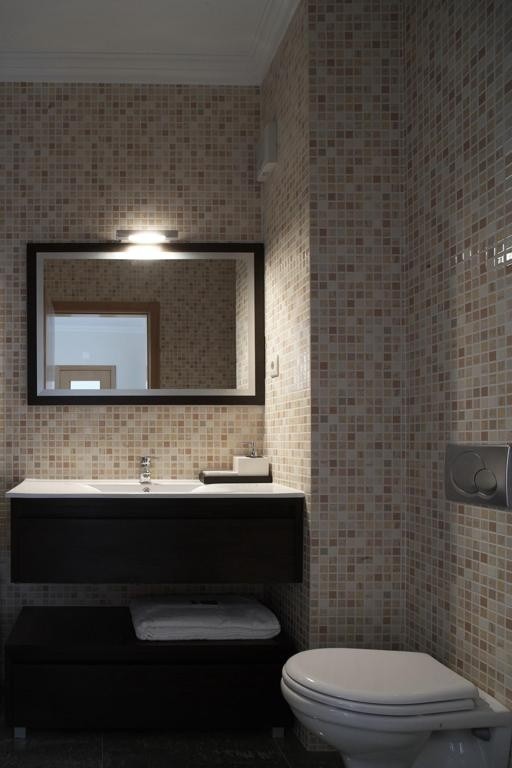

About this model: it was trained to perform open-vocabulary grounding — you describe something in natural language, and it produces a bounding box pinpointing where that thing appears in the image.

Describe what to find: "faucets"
[139,456,152,485]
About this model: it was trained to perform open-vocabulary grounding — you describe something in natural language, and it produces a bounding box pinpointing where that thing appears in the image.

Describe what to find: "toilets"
[279,644,512,768]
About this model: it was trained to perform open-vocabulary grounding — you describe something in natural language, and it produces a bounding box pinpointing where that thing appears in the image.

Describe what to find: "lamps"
[115,227,179,241]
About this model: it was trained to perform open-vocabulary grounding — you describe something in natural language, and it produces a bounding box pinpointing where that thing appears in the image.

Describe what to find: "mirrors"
[26,240,265,408]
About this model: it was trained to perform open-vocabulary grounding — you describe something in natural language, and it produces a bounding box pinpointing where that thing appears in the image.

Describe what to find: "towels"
[128,595,282,639]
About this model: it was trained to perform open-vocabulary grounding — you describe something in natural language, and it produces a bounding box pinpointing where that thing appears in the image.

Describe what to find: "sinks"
[87,482,204,495]
